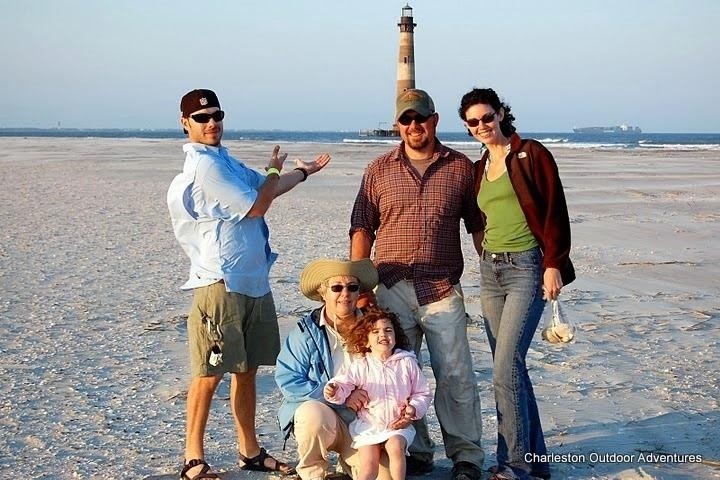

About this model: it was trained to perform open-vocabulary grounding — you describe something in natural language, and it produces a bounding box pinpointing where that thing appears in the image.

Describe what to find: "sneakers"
[452,461,481,480]
[406,456,435,475]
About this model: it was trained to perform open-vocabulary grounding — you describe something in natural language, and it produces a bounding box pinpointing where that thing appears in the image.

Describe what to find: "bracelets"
[294,168,308,182]
[266,168,280,178]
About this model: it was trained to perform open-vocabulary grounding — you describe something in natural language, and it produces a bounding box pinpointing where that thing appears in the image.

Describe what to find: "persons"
[350,89,484,480]
[323,309,432,480]
[167,89,331,480]
[459,88,577,480]
[274,257,391,480]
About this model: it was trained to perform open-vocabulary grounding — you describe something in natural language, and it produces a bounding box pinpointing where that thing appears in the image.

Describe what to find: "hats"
[301,258,379,303]
[395,89,435,120]
[180,89,220,134]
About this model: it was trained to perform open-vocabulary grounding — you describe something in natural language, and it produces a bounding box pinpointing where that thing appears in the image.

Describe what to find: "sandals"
[486,472,513,480]
[239,448,289,472]
[488,466,550,480]
[180,460,221,480]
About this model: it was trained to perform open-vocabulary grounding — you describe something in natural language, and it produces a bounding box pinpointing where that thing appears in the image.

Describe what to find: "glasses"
[399,113,432,125]
[189,110,224,123]
[327,283,360,292]
[466,112,497,127]
